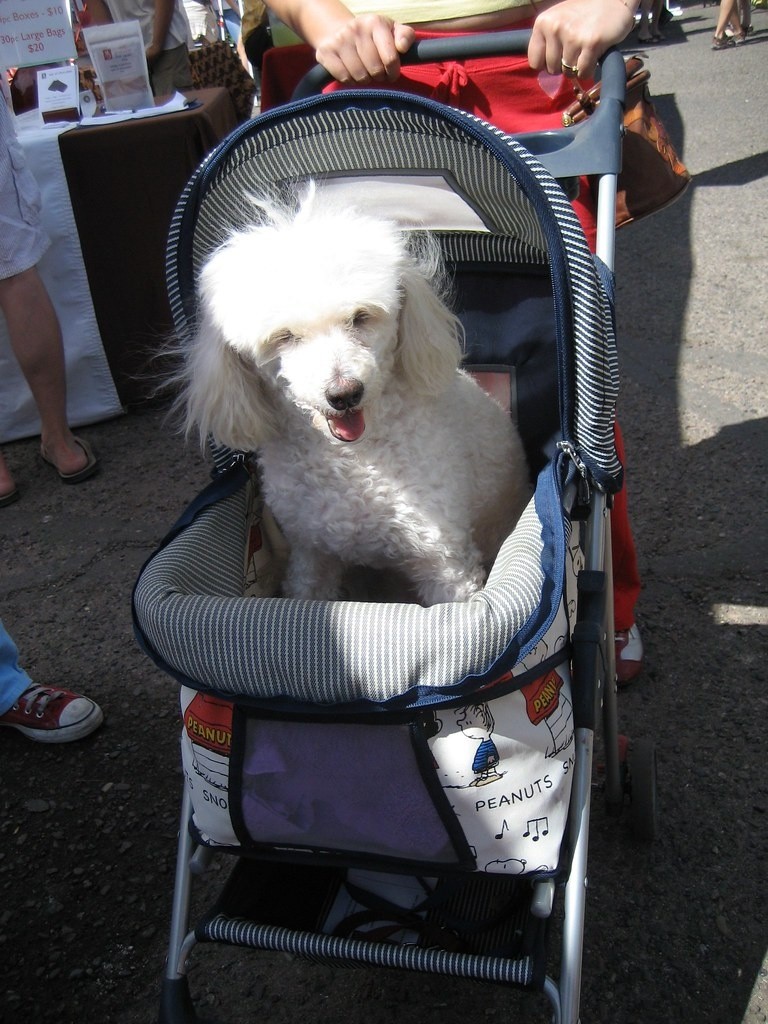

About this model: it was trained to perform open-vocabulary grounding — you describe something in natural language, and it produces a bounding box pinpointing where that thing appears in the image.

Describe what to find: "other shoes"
[615,624,645,688]
[731,25,753,45]
[712,33,729,49]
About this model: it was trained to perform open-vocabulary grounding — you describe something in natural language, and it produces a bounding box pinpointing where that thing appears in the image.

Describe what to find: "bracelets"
[617,0,636,33]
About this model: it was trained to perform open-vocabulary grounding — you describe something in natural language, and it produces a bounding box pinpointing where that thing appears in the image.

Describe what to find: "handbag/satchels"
[563,56,691,231]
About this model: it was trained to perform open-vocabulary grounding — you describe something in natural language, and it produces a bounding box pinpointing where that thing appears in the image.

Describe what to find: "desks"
[78,39,259,129]
[0,86,244,445]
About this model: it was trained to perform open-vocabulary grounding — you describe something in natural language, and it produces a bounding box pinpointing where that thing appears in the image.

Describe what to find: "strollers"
[129,24,660,1021]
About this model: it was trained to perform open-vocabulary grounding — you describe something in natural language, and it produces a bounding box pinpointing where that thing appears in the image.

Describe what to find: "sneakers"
[0,682,103,744]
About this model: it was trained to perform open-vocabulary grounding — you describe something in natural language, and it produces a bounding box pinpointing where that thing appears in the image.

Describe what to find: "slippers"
[0,490,18,507]
[636,33,664,44]
[41,435,96,482]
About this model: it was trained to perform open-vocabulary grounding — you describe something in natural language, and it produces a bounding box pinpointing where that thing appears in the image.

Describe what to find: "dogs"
[130,176,531,603]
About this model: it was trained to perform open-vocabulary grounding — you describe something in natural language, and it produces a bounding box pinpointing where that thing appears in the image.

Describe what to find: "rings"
[562,58,578,73]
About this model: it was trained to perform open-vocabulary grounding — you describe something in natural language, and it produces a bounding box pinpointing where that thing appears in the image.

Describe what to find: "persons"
[183,0,273,98]
[263,0,641,686]
[0,620,104,744]
[711,0,753,50]
[86,0,193,96]
[0,90,114,508]
[638,0,666,44]
